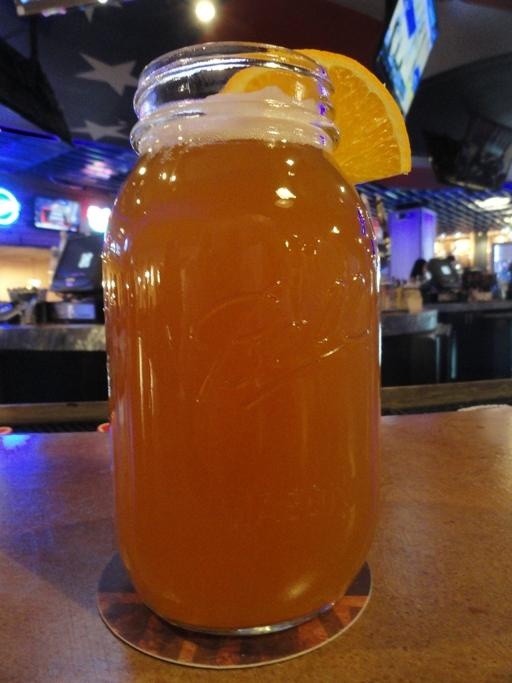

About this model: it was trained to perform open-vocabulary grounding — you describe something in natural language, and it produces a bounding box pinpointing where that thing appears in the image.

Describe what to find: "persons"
[404,258,429,289]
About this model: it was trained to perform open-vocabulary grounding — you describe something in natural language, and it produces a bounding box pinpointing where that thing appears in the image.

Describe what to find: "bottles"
[97,39,383,636]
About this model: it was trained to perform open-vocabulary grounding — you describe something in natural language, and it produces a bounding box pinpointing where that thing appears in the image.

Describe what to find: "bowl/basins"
[6,286,47,301]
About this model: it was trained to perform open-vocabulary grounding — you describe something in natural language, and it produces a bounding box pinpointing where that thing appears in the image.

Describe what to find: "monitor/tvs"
[368,0,441,119]
[48,234,101,299]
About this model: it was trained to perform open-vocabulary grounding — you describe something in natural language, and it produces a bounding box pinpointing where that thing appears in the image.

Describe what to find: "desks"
[378,309,440,383]
[424,302,512,383]
[1,322,107,403]
